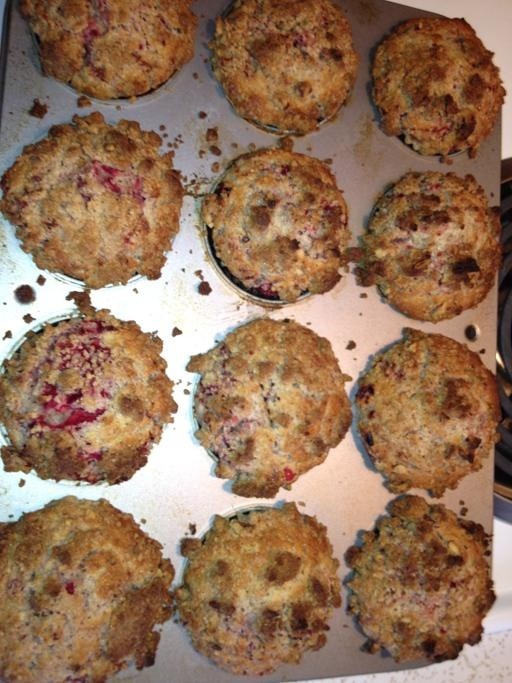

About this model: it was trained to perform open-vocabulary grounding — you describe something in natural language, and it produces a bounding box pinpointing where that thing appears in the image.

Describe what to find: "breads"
[0,1,508,682]
